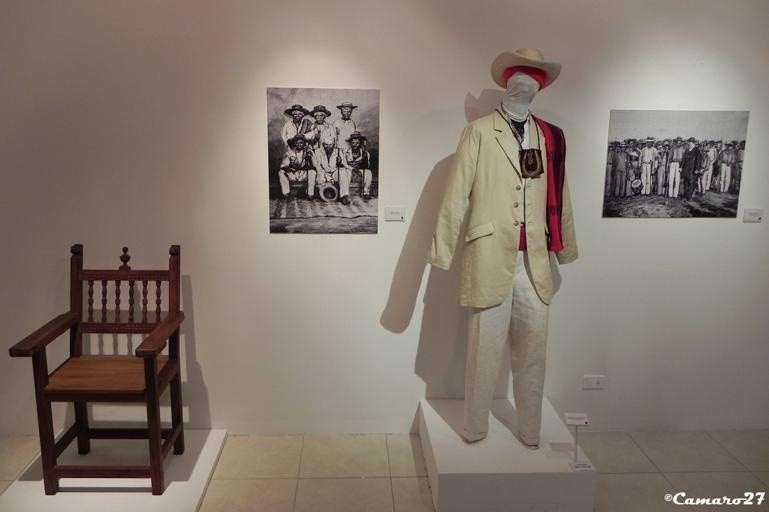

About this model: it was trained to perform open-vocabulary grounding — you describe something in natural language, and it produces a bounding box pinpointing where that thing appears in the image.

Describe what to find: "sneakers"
[363,194,372,201]
[339,196,351,205]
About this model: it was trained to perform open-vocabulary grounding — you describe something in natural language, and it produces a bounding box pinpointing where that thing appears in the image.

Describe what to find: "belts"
[607,159,744,165]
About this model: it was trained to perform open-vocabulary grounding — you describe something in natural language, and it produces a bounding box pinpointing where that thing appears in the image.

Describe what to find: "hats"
[287,133,311,150]
[317,183,339,203]
[344,131,367,142]
[308,105,331,117]
[336,101,358,110]
[283,104,309,117]
[608,136,734,149]
[488,47,564,90]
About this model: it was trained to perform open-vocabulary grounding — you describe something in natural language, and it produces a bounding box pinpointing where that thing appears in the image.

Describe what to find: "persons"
[606,134,747,202]
[276,99,373,208]
[427,43,579,450]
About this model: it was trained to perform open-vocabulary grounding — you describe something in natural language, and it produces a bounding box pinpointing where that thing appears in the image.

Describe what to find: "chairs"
[10,243,186,495]
